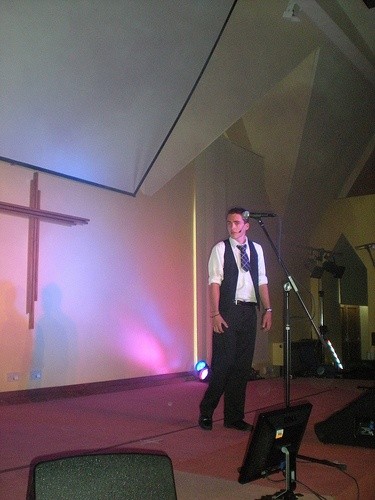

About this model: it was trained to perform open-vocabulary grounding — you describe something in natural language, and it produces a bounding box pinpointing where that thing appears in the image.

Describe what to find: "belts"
[237,301,257,306]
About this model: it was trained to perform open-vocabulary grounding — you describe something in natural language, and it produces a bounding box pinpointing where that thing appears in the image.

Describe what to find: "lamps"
[322,260,346,278]
[195,359,212,383]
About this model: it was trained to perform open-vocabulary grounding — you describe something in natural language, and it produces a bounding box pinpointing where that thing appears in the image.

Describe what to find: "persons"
[199,208,272,432]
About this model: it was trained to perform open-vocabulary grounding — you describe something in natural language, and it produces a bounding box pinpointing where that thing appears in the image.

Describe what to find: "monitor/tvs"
[239,401,312,499]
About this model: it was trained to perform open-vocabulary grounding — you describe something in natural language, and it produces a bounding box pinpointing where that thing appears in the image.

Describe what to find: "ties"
[236,244,251,272]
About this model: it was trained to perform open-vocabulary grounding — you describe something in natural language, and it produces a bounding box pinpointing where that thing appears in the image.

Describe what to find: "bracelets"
[265,308,272,312]
[211,314,219,318]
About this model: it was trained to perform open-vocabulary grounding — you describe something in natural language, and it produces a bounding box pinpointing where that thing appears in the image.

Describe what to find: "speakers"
[27,451,178,500]
[313,385,374,447]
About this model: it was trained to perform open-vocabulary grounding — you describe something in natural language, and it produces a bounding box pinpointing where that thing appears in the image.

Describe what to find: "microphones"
[242,210,277,220]
[355,242,375,251]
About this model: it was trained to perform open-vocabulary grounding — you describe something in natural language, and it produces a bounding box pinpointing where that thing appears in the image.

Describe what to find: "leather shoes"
[198,415,213,431]
[223,420,254,432]
